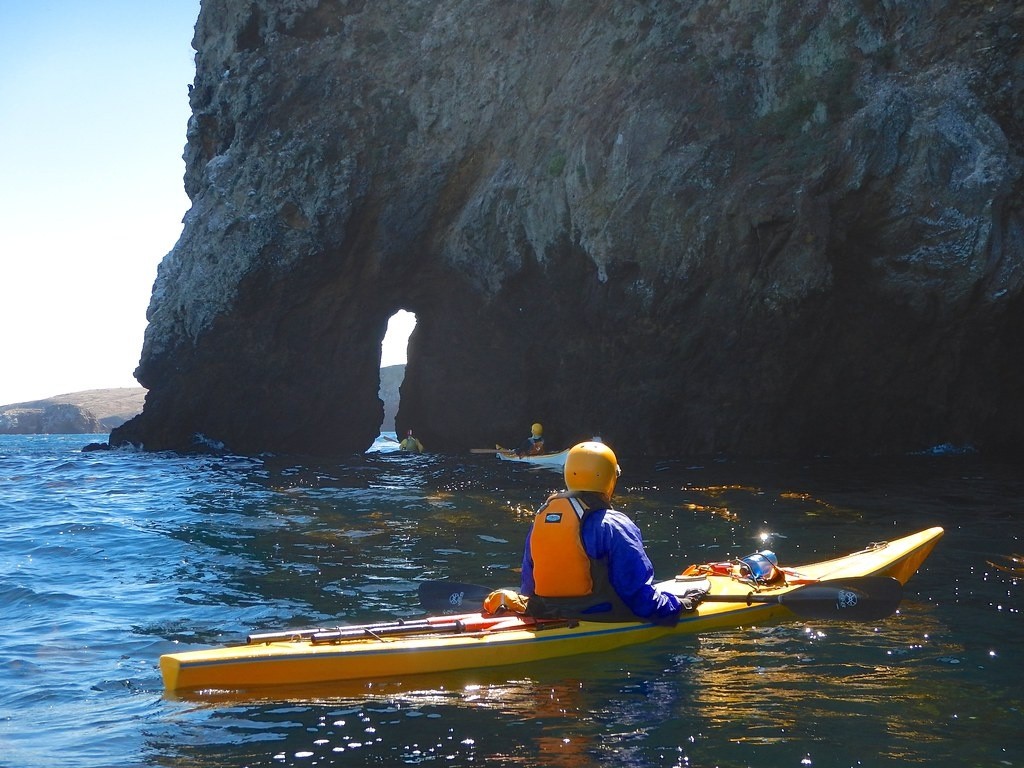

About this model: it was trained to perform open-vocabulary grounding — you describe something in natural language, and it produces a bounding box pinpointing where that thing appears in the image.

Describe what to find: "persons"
[399,430,424,453]
[495,422,546,458]
[482,441,708,627]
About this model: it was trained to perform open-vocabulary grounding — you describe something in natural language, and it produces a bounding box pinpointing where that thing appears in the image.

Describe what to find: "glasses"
[616,464,621,479]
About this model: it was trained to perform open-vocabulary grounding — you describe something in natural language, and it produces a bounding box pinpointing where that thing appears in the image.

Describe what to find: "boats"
[496,436,603,473]
[159,526,944,694]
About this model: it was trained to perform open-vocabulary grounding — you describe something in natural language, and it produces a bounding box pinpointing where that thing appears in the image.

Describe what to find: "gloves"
[679,589,707,613]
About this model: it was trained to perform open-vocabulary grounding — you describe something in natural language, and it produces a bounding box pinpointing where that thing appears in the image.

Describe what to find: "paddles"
[245,610,580,647]
[417,574,905,623]
[470,448,513,454]
[383,436,402,444]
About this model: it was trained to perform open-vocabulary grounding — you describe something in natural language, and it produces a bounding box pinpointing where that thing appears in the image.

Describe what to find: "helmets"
[407,429,415,436]
[532,423,543,436]
[564,441,619,503]
